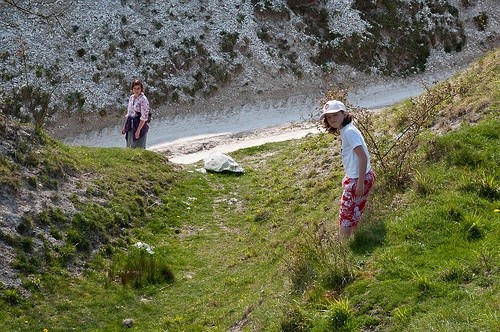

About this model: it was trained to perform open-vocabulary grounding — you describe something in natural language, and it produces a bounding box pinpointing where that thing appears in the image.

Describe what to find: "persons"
[319,100,376,243]
[120,80,152,149]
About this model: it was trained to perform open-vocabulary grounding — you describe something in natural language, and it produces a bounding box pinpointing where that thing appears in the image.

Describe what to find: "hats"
[320,100,346,120]
